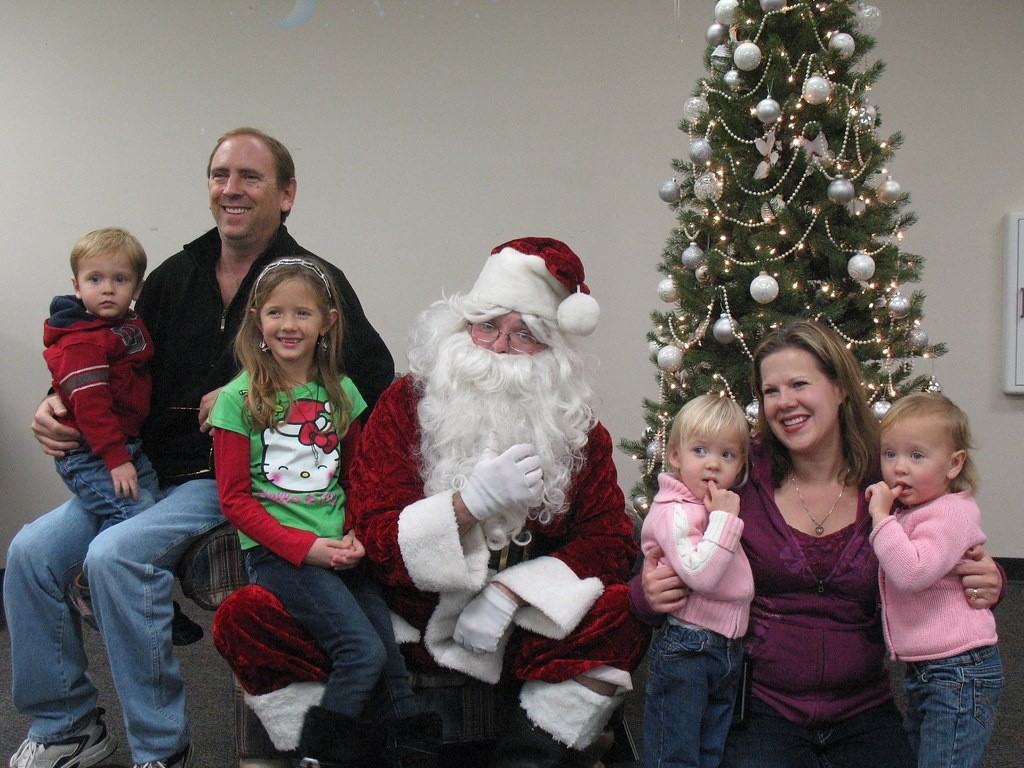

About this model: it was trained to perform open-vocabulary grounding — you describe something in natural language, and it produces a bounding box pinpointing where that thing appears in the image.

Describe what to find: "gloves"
[453,582,519,655]
[458,444,543,521]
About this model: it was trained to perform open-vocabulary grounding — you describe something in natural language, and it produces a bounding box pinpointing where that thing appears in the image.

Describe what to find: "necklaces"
[792,468,850,536]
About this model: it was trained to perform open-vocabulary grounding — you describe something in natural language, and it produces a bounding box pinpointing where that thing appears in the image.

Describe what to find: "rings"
[971,588,977,601]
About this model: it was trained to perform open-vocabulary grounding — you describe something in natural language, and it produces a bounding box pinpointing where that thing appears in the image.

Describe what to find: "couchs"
[174,502,648,768]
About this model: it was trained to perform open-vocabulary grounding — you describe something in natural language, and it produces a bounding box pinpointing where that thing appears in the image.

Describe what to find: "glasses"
[467,320,544,353]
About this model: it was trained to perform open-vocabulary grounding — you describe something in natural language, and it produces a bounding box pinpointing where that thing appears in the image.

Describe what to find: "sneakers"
[131,742,195,768]
[167,601,203,644]
[7,706,117,768]
[64,571,99,631]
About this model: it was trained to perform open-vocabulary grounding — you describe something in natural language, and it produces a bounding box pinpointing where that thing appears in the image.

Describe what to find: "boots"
[292,706,376,768]
[243,678,328,768]
[487,674,628,768]
[380,711,442,768]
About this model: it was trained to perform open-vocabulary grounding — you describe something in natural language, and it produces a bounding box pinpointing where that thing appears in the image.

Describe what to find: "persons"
[641,395,755,768]
[43,229,204,647]
[211,237,652,768]
[630,320,1008,768]
[205,257,444,768]
[3,128,395,768]
[865,392,1004,768]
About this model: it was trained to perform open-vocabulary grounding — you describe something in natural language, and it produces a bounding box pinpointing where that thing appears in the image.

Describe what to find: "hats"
[467,236,600,336]
